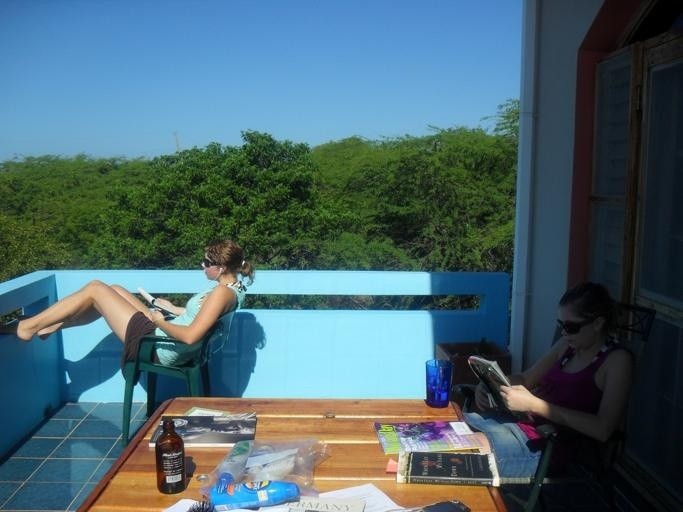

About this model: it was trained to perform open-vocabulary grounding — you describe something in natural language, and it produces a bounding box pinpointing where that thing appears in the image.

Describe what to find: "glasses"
[203,258,217,267]
[557,316,599,335]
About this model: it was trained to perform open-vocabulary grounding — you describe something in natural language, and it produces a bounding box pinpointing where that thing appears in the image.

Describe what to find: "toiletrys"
[215,440,254,492]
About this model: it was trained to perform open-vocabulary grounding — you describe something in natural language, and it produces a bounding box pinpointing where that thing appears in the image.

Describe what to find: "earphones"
[220,267,223,273]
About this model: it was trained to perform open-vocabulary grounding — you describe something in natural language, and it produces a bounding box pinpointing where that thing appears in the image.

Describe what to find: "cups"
[425,359,453,408]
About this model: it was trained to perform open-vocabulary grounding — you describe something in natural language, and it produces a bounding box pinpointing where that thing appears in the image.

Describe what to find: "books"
[373,420,473,456]
[395,449,500,489]
[467,353,529,421]
[137,287,176,315]
[148,412,258,448]
[384,430,494,475]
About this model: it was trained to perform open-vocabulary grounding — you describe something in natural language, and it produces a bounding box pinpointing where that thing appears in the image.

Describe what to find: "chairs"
[122,306,236,448]
[450,300,656,511]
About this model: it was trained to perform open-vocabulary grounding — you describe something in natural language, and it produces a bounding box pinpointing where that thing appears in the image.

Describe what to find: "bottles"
[155,420,186,495]
[209,441,299,512]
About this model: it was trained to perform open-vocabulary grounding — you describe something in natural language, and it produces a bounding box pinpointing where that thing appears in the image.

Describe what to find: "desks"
[75,396,508,511]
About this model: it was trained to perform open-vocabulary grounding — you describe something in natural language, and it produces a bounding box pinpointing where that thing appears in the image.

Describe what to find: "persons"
[419,456,464,475]
[161,415,256,440]
[463,281,637,490]
[0,239,256,371]
